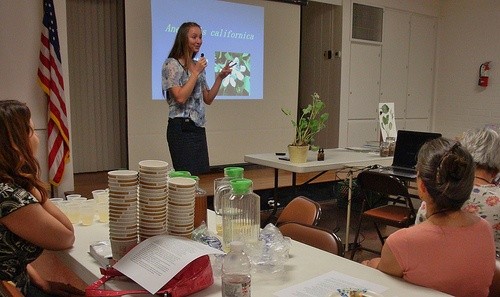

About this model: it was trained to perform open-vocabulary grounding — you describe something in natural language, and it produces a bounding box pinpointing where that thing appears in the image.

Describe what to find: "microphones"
[200,53,204,57]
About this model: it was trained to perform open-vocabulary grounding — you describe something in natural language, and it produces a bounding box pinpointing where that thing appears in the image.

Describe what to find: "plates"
[331,288,379,297]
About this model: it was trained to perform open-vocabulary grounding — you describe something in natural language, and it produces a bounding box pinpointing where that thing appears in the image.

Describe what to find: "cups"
[107,160,196,262]
[49,189,107,225]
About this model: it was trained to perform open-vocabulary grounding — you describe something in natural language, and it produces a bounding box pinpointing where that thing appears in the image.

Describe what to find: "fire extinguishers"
[478,60,492,87]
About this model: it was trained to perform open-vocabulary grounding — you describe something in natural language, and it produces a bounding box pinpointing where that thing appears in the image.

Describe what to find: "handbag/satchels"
[84,254,214,297]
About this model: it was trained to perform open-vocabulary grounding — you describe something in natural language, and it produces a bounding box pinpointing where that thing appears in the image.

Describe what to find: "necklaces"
[474,176,490,184]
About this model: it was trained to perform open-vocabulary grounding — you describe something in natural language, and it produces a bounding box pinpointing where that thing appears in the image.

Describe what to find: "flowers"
[281,92,329,152]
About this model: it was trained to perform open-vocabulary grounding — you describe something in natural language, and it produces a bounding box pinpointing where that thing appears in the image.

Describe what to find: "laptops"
[368,130,443,178]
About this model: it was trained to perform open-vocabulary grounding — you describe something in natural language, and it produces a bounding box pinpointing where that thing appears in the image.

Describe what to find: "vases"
[287,144,310,163]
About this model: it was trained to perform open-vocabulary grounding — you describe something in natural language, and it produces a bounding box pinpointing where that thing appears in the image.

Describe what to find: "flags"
[36,0,71,186]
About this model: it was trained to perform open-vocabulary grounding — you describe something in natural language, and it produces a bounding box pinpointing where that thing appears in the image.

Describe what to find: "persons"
[361,138,496,297]
[161,22,237,176]
[0,100,74,297]
[414,127,500,260]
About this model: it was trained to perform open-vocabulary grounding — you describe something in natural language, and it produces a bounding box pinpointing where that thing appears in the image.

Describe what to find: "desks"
[56,198,456,297]
[244,146,394,213]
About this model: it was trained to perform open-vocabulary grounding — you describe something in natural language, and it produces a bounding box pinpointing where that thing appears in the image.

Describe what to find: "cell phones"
[279,157,290,160]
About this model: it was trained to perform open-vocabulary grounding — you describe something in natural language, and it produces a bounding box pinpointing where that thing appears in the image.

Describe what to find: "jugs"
[169,170,208,230]
[213,178,261,248]
[213,167,244,234]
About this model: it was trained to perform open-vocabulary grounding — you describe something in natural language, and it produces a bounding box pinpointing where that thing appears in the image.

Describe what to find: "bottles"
[379,141,390,157]
[318,148,324,161]
[220,241,253,297]
[389,143,395,156]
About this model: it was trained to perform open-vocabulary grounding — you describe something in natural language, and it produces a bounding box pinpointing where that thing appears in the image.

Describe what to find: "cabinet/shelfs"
[347,12,439,147]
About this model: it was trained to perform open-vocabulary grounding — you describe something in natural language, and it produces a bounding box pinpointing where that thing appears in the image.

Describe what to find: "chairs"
[274,169,424,257]
